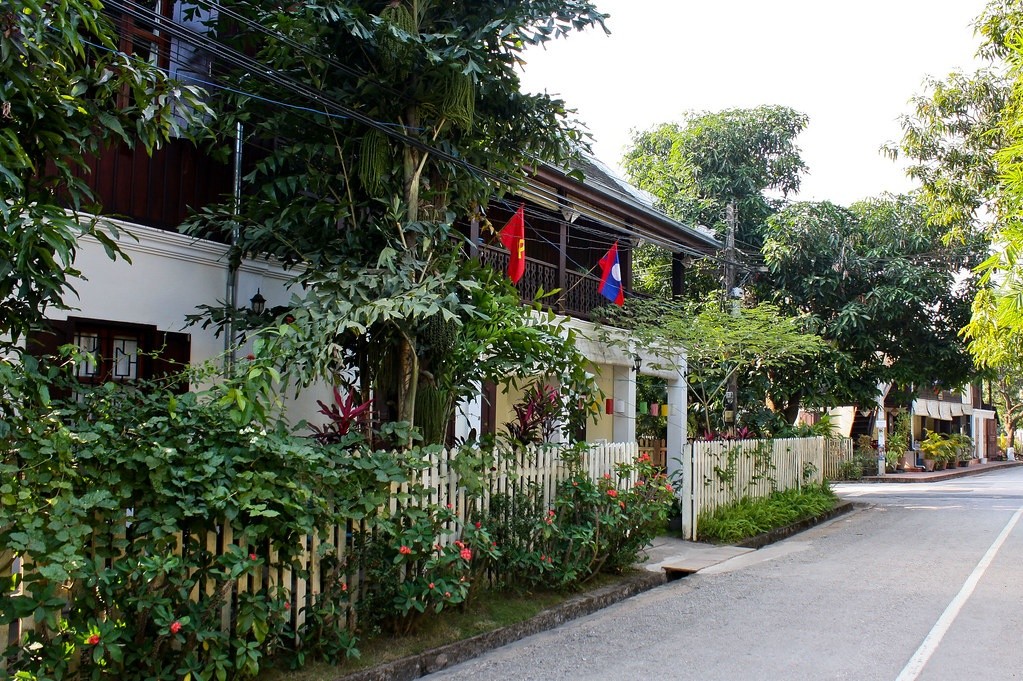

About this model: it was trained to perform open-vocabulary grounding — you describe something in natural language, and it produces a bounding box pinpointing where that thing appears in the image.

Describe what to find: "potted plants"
[845,434,908,479]
[918,428,974,471]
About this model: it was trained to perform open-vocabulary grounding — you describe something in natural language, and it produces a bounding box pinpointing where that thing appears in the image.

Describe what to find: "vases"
[981,457,987,464]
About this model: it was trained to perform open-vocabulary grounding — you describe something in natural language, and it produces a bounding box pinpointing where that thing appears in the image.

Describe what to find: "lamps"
[561,202,580,223]
[631,234,645,248]
[681,255,695,269]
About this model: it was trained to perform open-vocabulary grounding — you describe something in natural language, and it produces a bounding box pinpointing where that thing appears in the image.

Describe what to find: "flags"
[598,244,624,307]
[496,205,525,284]
